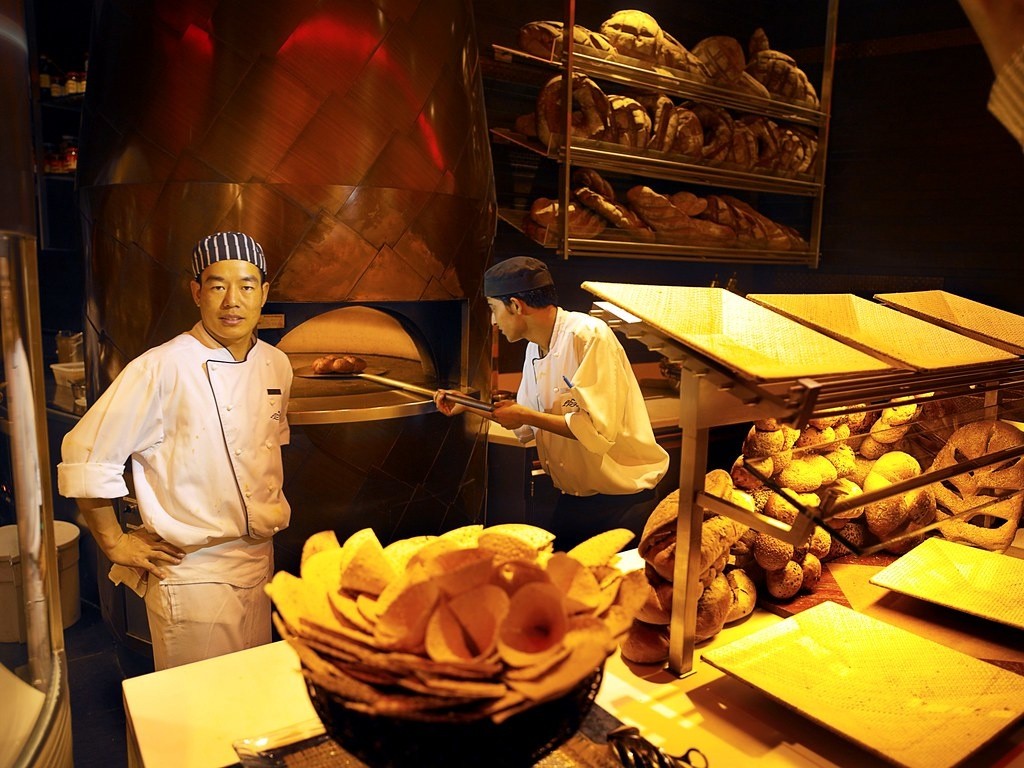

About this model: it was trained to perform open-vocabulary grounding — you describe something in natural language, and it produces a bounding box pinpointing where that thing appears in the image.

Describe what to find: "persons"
[58,230,295,671]
[433,257,672,553]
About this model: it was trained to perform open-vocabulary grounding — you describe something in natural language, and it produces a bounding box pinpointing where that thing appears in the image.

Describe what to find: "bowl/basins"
[71,384,86,406]
[50,362,85,388]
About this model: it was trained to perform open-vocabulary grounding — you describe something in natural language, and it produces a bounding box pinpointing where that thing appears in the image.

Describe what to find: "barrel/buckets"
[54,330,84,363]
[0,520,81,645]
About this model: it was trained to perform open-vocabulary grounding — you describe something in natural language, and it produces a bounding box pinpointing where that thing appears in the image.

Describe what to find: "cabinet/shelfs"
[492,2,840,270]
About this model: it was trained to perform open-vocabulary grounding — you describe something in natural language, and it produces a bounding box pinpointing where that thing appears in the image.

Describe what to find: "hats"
[483,257,552,297]
[192,230,268,282]
